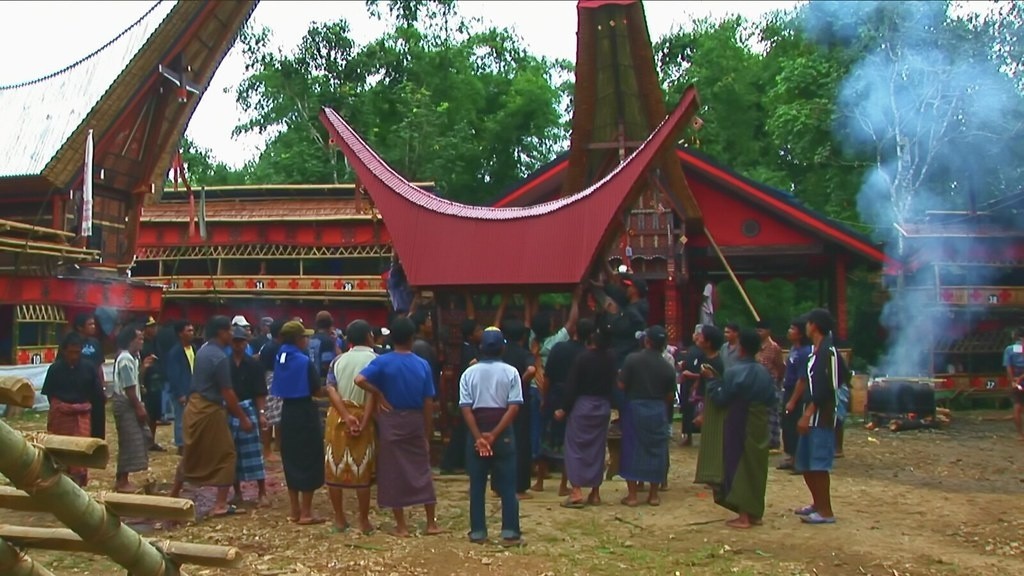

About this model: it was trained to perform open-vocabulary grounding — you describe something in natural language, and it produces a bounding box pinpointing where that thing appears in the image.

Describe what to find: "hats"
[481,326,503,357]
[623,274,648,297]
[645,325,668,346]
[279,321,315,341]
[232,315,250,326]
[233,327,249,340]
[315,310,333,321]
[139,314,156,327]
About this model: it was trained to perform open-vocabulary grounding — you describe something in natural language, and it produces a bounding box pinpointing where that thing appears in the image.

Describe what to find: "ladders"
[0,373,243,576]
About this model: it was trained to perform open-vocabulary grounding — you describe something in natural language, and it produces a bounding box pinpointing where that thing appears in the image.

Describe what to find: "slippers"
[588,495,601,506]
[297,515,326,524]
[795,504,818,515]
[560,497,585,508]
[800,512,835,523]
[213,504,246,514]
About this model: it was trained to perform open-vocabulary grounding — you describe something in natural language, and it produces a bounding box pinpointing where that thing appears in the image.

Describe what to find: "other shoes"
[147,443,167,451]
[500,539,528,547]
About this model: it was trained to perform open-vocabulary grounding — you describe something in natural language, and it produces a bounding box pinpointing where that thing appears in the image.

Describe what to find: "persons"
[1001,327,1024,441]
[39,248,851,543]
